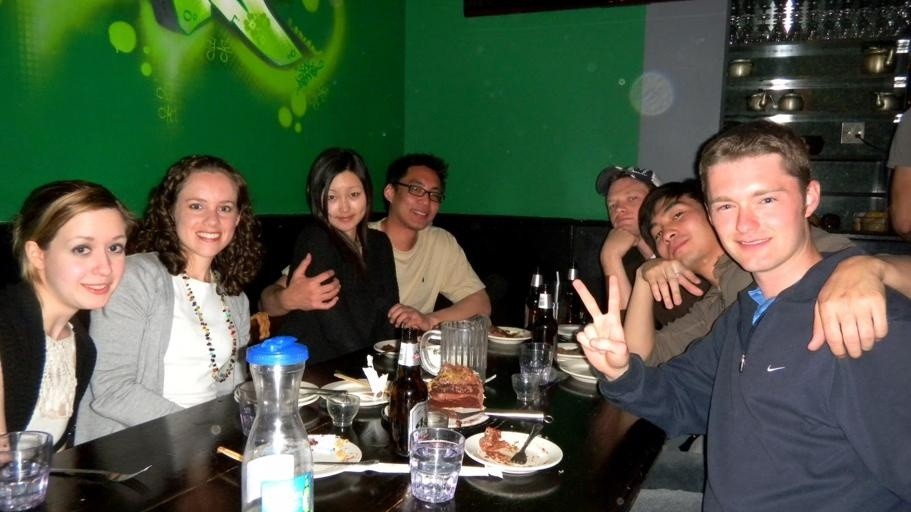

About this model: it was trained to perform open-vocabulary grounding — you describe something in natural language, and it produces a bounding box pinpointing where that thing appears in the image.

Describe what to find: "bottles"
[240,336,315,512]
[392,324,430,458]
[527,259,585,372]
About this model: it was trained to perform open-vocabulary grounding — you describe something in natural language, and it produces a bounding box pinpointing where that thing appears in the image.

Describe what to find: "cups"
[419,318,490,386]
[420,410,450,430]
[513,373,540,404]
[2,429,54,511]
[409,427,466,504]
[325,392,360,428]
[521,342,555,386]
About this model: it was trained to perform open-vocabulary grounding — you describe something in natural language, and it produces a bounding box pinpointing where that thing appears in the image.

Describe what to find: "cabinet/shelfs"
[719,0,911,253]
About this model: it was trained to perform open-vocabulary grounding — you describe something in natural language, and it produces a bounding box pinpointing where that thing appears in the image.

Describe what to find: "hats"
[596,165,662,196]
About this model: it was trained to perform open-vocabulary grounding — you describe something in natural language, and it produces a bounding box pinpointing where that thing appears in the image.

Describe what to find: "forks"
[56,462,155,483]
[511,421,544,466]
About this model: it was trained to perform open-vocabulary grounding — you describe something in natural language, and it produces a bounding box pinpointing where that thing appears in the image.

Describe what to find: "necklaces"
[181,267,236,383]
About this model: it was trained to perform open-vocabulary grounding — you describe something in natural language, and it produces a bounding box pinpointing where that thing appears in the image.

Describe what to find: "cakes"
[479,427,508,452]
[427,362,486,420]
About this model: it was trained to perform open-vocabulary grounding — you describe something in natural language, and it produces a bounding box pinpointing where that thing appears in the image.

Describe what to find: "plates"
[380,402,492,427]
[488,325,532,346]
[373,336,403,360]
[487,322,534,347]
[554,320,605,386]
[234,379,322,411]
[463,430,564,476]
[418,400,491,428]
[308,433,364,479]
[319,377,395,408]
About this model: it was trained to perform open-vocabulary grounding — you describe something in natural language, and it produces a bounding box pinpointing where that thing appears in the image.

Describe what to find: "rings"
[676,272,681,278]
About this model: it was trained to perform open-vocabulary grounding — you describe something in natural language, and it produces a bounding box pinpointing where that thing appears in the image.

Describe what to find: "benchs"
[3,213,621,351]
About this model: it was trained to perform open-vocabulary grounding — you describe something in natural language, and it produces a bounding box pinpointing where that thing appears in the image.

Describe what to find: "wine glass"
[730,0,911,44]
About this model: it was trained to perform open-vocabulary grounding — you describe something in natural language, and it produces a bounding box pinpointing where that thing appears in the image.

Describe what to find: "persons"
[272,147,403,366]
[571,119,911,512]
[259,154,493,331]
[622,179,911,370]
[75,153,251,446]
[888,110,911,240]
[0,180,135,472]
[595,164,663,312]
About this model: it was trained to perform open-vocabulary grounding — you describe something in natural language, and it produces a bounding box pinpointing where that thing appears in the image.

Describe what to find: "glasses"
[398,181,445,203]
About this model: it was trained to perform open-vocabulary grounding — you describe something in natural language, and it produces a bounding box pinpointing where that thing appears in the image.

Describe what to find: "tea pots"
[728,56,754,80]
[747,88,807,113]
[859,45,895,76]
[873,85,896,117]
